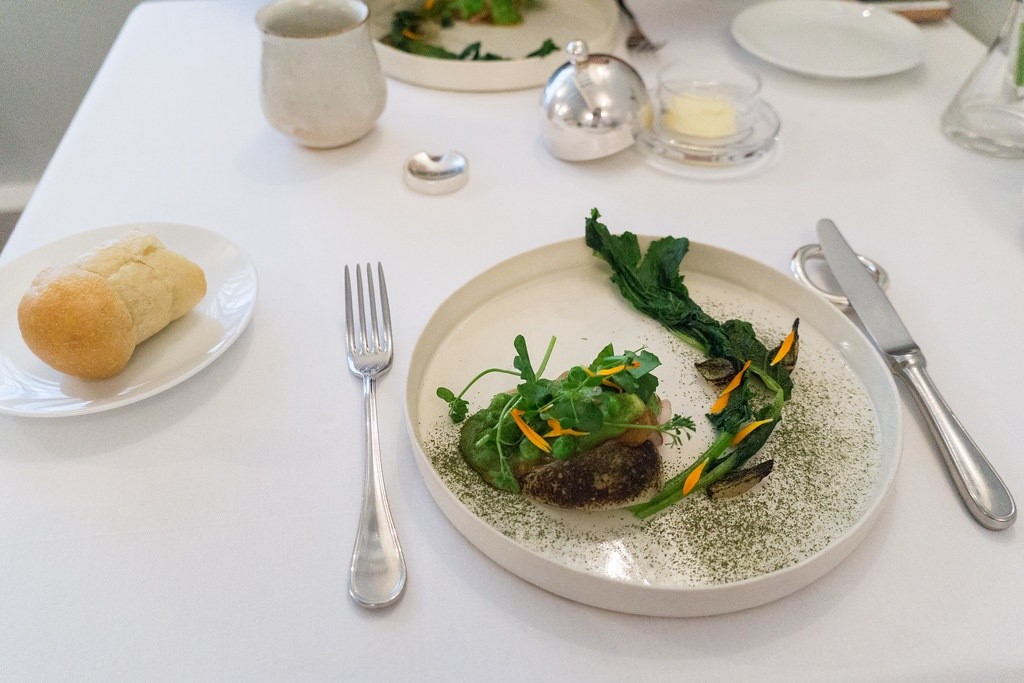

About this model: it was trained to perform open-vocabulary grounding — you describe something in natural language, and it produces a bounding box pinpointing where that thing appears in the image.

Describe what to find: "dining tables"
[0,0,1024,683]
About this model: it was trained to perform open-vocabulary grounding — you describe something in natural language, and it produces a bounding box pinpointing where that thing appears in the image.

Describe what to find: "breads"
[17,229,207,378]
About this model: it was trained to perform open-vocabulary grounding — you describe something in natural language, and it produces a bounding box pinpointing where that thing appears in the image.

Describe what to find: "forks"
[344,261,407,610]
[614,0,669,56]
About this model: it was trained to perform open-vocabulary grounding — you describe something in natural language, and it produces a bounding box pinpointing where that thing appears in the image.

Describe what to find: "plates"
[731,0,929,82]
[404,234,904,619]
[0,221,258,419]
[633,88,782,178]
[363,0,622,93]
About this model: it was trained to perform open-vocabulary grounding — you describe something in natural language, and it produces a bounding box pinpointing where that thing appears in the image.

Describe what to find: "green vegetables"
[435,207,797,521]
[388,0,560,61]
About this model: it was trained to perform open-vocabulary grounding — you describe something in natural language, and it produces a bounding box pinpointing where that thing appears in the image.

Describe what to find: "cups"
[940,0,1024,162]
[253,0,387,150]
[656,61,763,147]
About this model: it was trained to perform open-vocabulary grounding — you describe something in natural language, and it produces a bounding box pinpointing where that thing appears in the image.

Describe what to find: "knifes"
[814,218,1018,531]
